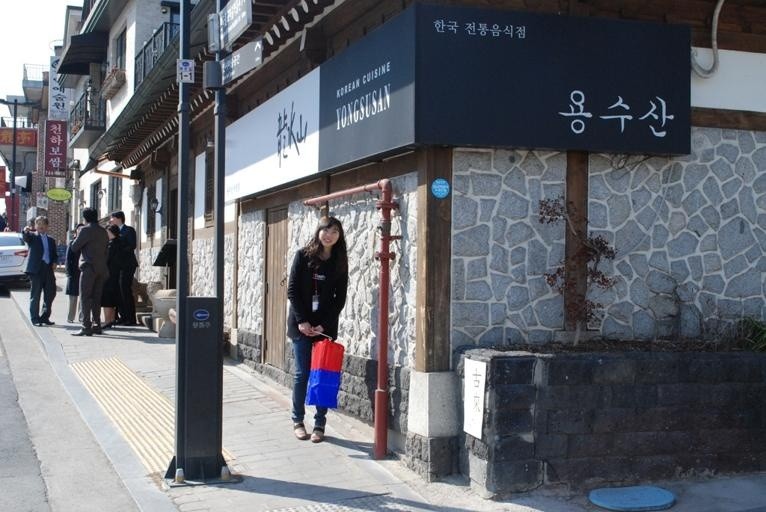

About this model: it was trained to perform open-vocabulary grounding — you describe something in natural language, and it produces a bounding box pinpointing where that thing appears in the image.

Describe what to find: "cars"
[0,232,32,284]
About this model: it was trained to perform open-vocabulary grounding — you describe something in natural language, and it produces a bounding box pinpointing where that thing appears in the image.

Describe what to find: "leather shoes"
[294,423,307,440]
[67,320,136,336]
[34,320,54,326]
[311,427,324,443]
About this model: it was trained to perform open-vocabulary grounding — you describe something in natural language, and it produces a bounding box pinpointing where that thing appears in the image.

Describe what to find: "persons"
[22,215,56,326]
[286,215,349,443]
[69,206,109,337]
[3,225,14,232]
[108,210,139,326]
[0,215,8,232]
[99,223,122,330]
[65,223,86,322]
